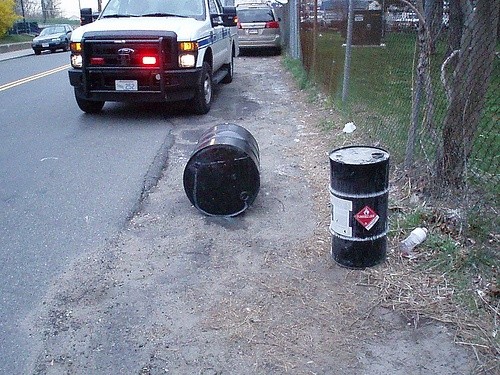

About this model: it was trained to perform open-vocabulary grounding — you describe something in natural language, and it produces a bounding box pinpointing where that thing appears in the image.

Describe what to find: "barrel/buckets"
[330,144,392,272]
[183,123,261,219]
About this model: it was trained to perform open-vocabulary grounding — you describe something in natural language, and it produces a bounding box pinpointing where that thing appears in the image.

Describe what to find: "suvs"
[67,0,237,113]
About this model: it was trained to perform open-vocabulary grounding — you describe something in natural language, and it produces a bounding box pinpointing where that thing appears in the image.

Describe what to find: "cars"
[30,24,72,54]
[300,2,419,32]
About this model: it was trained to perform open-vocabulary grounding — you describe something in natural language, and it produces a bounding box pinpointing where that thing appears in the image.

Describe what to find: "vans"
[234,3,282,55]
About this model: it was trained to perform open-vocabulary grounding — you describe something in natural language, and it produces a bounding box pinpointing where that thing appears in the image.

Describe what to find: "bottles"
[399,227,429,254]
